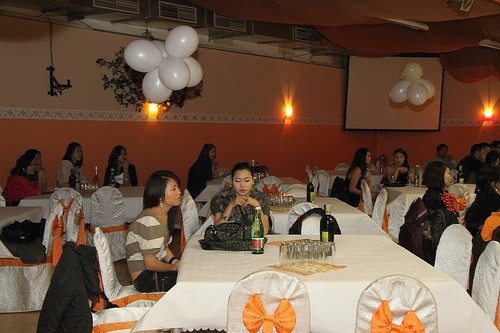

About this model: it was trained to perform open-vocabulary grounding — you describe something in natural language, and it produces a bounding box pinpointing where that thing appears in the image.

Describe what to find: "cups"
[279,239,336,261]
[268,196,294,208]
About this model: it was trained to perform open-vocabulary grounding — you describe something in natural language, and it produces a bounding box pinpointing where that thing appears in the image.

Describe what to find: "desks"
[312,170,384,194]
[385,186,428,214]
[199,198,393,242]
[130,236,500,333]
[18,186,143,223]
[0,206,45,257]
[195,175,309,202]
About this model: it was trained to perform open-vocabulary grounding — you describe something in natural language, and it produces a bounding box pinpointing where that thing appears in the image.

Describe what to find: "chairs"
[0,163,500,333]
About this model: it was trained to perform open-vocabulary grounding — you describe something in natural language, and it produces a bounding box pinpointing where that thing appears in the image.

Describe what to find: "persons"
[125,170,180,293]
[187,143,227,224]
[416,138,500,265]
[345,147,377,207]
[385,148,412,187]
[56,142,83,188]
[210,162,272,236]
[103,145,138,188]
[2,148,47,207]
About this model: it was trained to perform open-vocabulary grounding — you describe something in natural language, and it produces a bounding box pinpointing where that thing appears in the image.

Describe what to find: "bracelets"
[254,206,261,211]
[169,256,178,264]
[122,168,129,171]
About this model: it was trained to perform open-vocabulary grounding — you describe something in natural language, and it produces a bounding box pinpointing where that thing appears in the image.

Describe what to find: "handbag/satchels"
[134,270,177,293]
[199,222,251,251]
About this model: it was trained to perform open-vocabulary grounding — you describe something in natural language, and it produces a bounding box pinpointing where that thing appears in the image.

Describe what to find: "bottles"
[251,206,265,255]
[414,165,419,187]
[250,160,258,176]
[380,157,384,173]
[69,168,76,190]
[307,175,315,203]
[457,166,464,184]
[319,204,334,242]
[92,165,100,193]
[109,168,116,187]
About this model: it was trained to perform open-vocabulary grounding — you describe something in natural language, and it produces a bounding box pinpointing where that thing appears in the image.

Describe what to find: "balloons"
[388,62,436,106]
[124,26,203,103]
